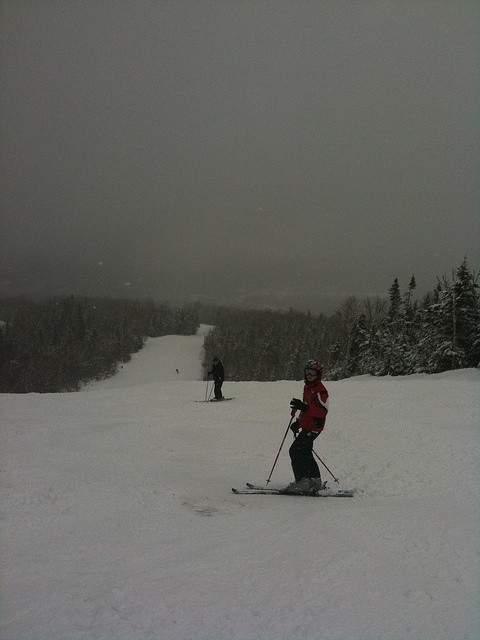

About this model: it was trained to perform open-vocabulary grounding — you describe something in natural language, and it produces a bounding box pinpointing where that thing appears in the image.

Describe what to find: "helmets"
[303,360,323,380]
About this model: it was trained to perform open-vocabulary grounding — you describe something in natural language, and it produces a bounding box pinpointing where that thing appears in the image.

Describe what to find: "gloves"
[290,398,308,411]
[290,420,300,433]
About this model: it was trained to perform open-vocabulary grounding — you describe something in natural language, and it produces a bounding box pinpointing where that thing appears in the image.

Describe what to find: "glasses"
[304,368,318,375]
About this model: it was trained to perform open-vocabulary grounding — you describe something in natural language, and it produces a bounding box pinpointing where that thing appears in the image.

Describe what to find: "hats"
[213,358,219,363]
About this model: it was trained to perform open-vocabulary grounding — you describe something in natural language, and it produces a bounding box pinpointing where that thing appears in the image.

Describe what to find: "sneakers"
[311,478,322,492]
[285,478,312,493]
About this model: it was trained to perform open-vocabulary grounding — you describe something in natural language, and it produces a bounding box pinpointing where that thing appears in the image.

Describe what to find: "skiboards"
[193,397,235,401]
[232,483,361,496]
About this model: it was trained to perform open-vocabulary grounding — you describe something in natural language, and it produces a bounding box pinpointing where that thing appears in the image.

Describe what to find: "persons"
[208,358,224,399]
[289,359,329,489]
